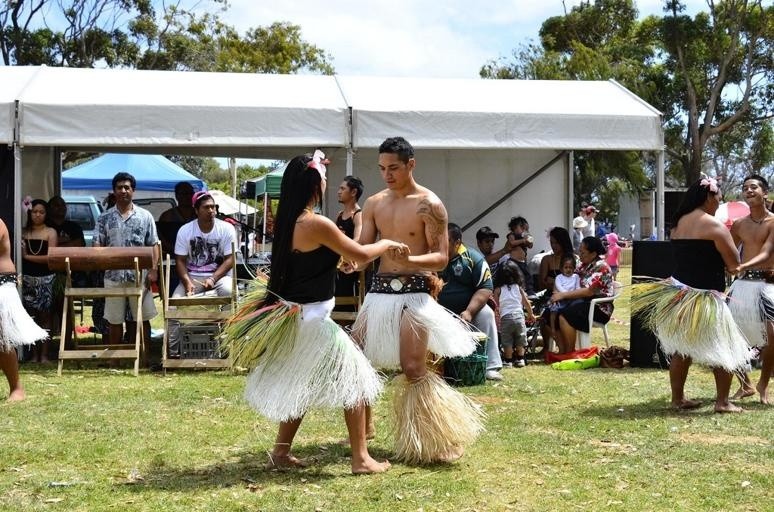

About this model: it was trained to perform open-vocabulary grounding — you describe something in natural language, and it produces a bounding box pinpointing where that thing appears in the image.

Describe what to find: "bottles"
[521,232,532,242]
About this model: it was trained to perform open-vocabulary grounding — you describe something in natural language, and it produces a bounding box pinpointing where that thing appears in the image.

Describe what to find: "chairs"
[546,281,621,353]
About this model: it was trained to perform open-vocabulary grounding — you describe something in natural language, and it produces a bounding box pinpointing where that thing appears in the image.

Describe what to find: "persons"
[264,149,408,476]
[669,177,744,415]
[1,221,48,403]
[341,137,465,466]
[169,190,238,358]
[729,173,774,405]
[435,204,623,382]
[335,176,364,326]
[91,172,159,369]
[156,182,198,296]
[24,195,86,363]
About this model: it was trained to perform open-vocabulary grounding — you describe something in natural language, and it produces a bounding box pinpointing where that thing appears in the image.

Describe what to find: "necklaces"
[748,213,769,225]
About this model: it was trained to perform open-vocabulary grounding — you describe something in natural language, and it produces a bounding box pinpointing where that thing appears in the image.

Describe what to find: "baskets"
[179,324,219,359]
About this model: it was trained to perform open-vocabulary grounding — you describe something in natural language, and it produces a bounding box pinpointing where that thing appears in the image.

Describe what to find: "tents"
[62,153,207,221]
[208,190,259,216]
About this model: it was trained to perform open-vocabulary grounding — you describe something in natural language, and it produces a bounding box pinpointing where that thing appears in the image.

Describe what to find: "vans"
[61,195,178,245]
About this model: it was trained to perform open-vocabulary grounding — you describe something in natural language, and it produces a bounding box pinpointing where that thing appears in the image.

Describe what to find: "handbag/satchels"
[600,346,625,369]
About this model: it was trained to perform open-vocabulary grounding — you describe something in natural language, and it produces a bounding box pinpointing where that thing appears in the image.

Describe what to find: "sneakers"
[486,369,502,380]
[514,359,525,367]
[503,361,512,368]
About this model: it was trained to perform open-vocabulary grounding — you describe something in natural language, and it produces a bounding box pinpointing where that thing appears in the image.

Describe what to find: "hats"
[606,233,618,243]
[586,206,600,214]
[192,191,210,207]
[476,226,499,238]
[573,217,588,228]
